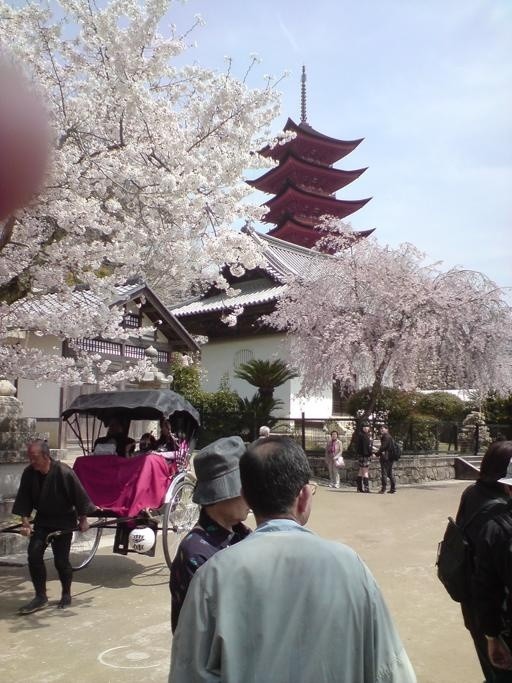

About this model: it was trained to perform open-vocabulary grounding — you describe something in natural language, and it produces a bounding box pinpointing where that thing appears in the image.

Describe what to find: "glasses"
[305,483,319,494]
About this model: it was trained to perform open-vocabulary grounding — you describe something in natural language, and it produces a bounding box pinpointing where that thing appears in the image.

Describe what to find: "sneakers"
[18,598,49,613]
[56,594,71,609]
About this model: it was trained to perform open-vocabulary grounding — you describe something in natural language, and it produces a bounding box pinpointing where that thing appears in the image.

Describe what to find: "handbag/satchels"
[334,456,345,467]
[390,442,402,461]
[436,496,507,599]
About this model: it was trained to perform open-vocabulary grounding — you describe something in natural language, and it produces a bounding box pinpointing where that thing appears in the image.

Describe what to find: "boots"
[363,477,369,492]
[357,476,364,493]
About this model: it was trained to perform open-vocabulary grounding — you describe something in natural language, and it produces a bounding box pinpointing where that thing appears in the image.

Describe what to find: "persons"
[354,419,374,493]
[169,436,262,634]
[0,43,55,227]
[11,437,98,617]
[257,424,272,438]
[153,413,179,451]
[138,429,157,450]
[91,412,136,456]
[324,428,344,488]
[166,435,419,683]
[451,438,512,683]
[373,423,397,494]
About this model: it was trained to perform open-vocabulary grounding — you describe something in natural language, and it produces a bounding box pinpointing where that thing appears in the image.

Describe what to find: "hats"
[498,459,511,486]
[193,436,248,505]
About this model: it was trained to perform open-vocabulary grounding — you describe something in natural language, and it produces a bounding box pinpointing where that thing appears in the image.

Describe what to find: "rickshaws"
[0,390,201,569]
